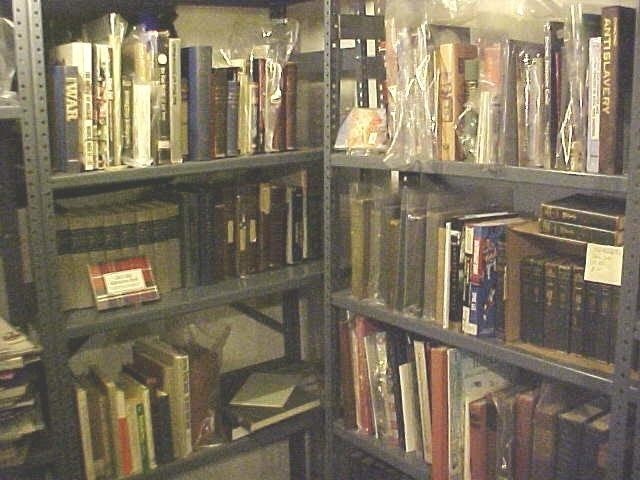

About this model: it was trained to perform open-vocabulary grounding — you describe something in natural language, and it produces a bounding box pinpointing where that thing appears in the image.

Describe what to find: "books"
[334,5,634,174]
[75,338,322,479]
[1,166,321,308]
[350,192,627,361]
[1,318,46,468]
[338,310,612,480]
[46,33,322,173]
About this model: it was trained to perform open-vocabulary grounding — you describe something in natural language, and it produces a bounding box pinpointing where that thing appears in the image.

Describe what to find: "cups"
[345,123,371,158]
